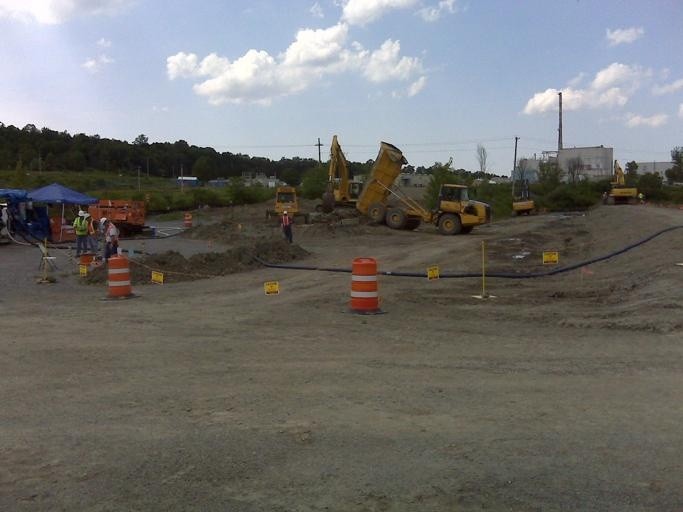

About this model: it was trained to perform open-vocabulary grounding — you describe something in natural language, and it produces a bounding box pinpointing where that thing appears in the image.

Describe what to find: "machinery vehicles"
[266,187,310,225]
[88,198,146,234]
[321,136,362,214]
[602,160,638,205]
[355,141,492,234]
[512,178,534,215]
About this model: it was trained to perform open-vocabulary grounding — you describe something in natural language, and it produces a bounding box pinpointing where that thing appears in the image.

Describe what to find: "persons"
[280,211,295,243]
[637,192,646,205]
[85,213,101,254]
[99,217,120,262]
[73,210,89,258]
[600,190,609,205]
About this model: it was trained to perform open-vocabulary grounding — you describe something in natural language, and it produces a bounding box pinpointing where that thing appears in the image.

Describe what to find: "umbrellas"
[26,182,98,241]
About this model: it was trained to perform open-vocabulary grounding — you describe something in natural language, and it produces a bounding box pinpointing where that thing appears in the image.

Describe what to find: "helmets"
[283,210,288,214]
[101,218,107,225]
[78,211,91,220]
